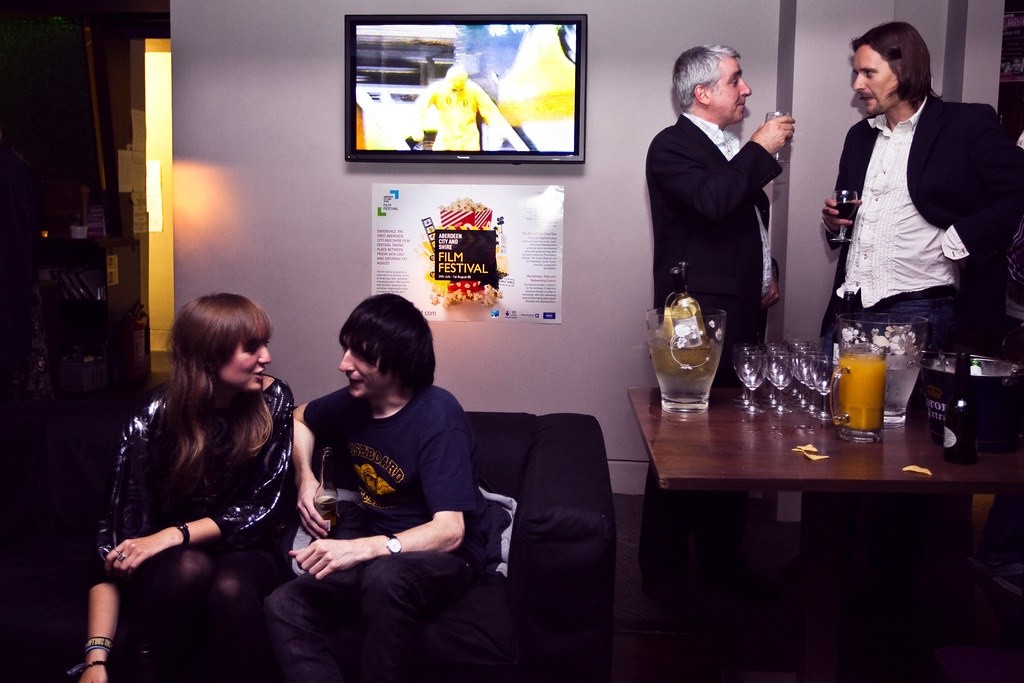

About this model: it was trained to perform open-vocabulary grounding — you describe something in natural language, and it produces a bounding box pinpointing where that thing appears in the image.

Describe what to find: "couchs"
[0,398,618,683]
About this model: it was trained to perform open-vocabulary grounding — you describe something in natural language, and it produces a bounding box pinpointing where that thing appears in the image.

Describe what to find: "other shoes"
[647,570,685,610]
[700,558,782,607]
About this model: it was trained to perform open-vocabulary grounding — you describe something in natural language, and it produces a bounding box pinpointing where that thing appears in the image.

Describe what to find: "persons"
[408,64,529,152]
[263,293,492,683]
[819,21,1024,617]
[78,294,294,683]
[639,47,795,634]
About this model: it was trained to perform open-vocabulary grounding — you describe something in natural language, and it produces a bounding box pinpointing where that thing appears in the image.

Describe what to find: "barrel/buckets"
[917,348,1023,452]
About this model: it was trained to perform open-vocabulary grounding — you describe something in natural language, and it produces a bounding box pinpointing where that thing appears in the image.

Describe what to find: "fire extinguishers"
[121,298,147,380]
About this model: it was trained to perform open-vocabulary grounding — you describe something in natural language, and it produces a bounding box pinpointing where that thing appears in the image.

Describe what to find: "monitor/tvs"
[344,14,588,164]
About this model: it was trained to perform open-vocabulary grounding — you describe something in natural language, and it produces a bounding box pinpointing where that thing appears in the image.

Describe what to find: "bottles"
[943,351,977,464]
[669,267,715,377]
[313,446,339,535]
[661,262,692,340]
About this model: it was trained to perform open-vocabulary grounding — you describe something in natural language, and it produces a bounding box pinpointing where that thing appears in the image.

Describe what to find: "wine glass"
[731,340,832,422]
[766,111,792,164]
[829,189,860,243]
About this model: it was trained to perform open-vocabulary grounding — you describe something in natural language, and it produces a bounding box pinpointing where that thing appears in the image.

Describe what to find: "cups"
[834,311,929,429]
[647,308,726,416]
[830,346,887,443]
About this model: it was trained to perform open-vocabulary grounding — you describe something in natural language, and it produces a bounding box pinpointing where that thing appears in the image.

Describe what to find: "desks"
[627,386,1024,683]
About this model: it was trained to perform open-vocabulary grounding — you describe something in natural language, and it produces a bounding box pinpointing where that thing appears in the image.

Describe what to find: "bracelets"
[80,661,106,673]
[84,636,113,653]
[174,522,190,544]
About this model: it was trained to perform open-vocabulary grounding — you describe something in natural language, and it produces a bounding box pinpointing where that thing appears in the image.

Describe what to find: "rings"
[118,554,125,562]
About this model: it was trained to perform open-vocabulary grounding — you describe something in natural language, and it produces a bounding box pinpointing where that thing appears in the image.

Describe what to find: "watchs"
[385,534,402,555]
